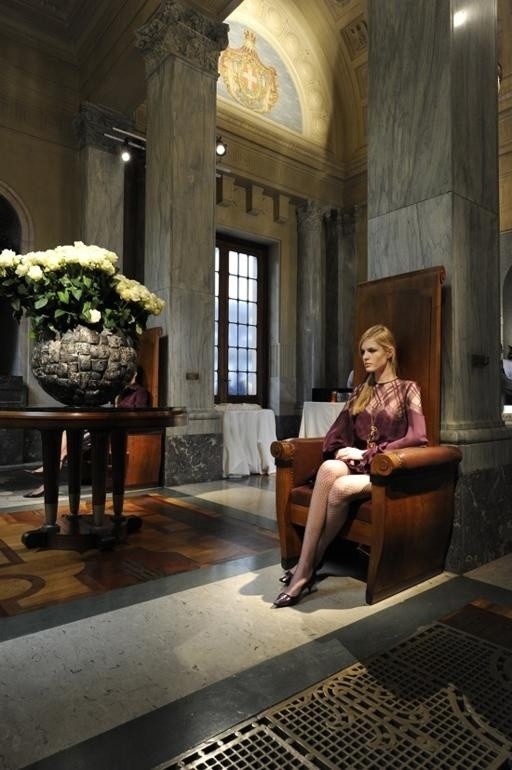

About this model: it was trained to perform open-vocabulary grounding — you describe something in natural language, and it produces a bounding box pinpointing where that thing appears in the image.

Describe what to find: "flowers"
[1,241,166,344]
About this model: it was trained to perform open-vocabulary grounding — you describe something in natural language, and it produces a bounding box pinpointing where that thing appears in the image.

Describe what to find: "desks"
[1,404,190,554]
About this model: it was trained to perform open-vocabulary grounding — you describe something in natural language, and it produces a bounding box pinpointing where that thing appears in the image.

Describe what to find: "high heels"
[280,559,325,583]
[273,570,314,607]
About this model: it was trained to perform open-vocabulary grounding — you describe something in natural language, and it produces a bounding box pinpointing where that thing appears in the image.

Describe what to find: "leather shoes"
[24,461,63,475]
[23,489,44,498]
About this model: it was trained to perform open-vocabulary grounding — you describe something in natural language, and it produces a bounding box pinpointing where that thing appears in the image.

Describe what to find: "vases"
[28,320,139,406]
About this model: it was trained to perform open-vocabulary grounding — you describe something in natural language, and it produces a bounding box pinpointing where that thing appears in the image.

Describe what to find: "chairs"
[268,263,461,605]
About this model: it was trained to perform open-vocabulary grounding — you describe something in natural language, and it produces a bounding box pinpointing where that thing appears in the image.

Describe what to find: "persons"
[273,323,429,608]
[23,364,150,498]
